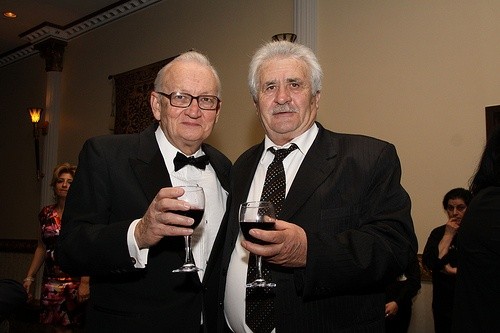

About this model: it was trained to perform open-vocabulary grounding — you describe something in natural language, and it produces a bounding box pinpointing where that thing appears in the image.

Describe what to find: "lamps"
[29,107,48,182]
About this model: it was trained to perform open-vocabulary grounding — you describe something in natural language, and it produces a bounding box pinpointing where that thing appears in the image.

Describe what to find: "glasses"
[155,91,221,110]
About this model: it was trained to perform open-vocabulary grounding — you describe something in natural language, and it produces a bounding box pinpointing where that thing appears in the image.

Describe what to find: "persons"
[386,222,422,333]
[24,163,89,333]
[222,40,411,333]
[55,51,232,333]
[455,122,500,332]
[422,188,472,333]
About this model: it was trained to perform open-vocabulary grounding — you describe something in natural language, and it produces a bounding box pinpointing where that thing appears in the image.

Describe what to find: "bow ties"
[173,152,210,172]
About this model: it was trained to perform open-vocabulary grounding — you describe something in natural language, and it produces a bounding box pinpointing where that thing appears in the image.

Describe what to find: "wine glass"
[164,185,206,273]
[238,201,278,288]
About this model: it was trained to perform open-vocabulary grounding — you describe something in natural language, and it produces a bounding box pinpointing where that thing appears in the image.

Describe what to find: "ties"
[245,143,298,333]
